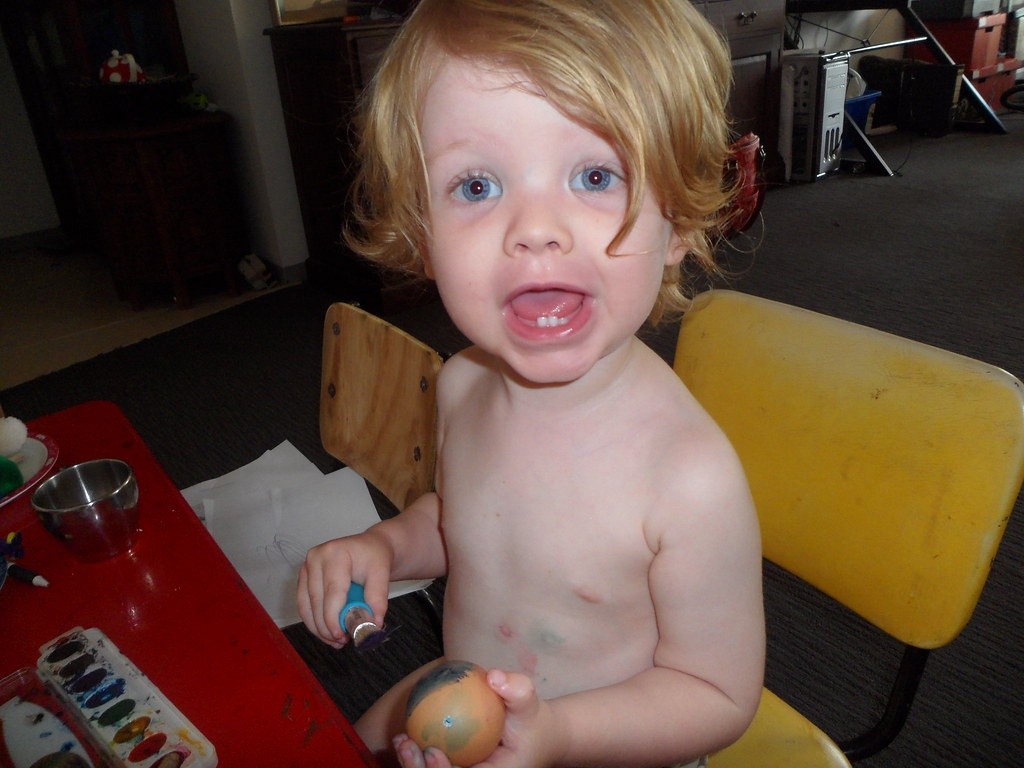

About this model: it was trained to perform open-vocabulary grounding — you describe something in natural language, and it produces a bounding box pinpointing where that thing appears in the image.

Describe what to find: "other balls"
[407,659,506,767]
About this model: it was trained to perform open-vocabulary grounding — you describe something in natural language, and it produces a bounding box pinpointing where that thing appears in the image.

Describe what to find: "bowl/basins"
[30,458,140,561]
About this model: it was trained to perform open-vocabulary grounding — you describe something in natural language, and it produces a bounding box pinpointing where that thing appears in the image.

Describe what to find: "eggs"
[405,660,504,766]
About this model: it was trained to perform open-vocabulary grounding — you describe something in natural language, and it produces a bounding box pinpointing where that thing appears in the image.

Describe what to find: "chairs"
[673,291,1024,768]
[318,301,442,645]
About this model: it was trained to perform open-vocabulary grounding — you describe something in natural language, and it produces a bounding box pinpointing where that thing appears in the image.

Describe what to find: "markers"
[6,561,50,588]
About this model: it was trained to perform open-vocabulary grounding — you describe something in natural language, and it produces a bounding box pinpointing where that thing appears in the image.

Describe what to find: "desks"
[41,109,253,312]
[0,399,376,768]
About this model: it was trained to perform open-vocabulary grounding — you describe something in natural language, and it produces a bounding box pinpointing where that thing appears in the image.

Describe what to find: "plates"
[0,432,60,508]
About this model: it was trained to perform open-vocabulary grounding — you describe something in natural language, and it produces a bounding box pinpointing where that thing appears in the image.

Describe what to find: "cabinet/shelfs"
[263,0,786,282]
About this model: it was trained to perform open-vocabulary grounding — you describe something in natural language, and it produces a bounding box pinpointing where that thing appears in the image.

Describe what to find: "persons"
[297,0,766,768]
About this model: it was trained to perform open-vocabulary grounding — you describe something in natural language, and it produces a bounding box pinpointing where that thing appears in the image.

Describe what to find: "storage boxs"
[956,57,1023,115]
[842,89,882,152]
[902,12,1007,68]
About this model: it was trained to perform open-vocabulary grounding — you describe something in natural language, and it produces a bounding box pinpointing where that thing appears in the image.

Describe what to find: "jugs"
[98,50,145,84]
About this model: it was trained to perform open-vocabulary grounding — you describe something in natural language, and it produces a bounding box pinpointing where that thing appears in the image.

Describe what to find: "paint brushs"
[339,581,389,657]
